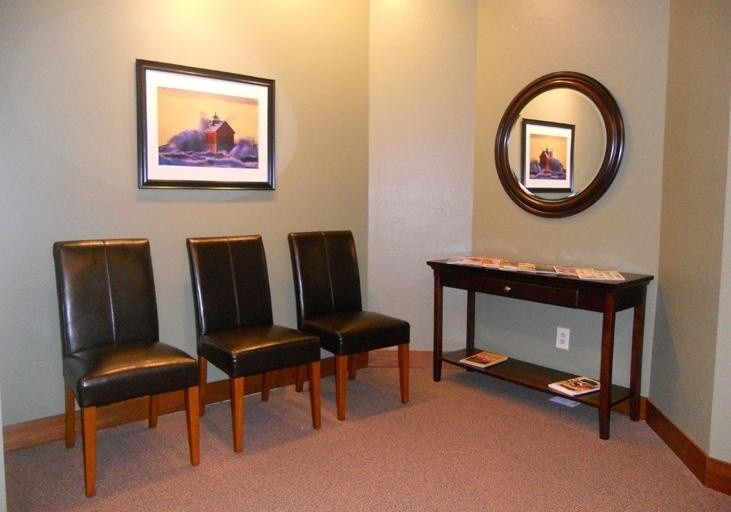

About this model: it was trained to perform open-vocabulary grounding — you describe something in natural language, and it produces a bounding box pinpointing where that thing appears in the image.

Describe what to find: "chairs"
[52,238,201,498]
[285,230,412,422]
[186,233,323,453]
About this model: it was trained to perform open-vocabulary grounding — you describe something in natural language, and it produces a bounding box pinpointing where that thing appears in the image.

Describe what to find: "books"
[498,258,521,271]
[457,351,510,370]
[446,257,464,264]
[547,375,603,397]
[551,263,593,277]
[461,256,483,267]
[574,267,626,281]
[516,261,539,273]
[480,256,504,270]
[532,262,561,277]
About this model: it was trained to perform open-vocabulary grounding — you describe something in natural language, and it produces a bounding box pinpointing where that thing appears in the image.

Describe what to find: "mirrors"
[494,72,625,219]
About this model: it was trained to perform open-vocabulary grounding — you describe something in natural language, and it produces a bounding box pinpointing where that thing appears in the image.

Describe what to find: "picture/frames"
[520,118,575,194]
[133,57,277,192]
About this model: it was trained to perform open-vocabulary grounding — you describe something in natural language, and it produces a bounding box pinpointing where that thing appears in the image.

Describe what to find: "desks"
[423,255,654,442]
